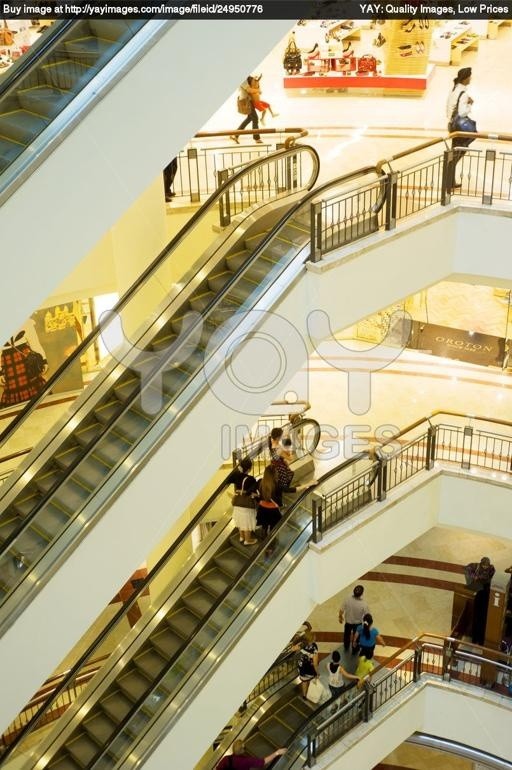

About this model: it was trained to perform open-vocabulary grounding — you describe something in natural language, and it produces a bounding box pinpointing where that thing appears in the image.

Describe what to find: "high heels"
[343,42,354,58]
[307,43,320,59]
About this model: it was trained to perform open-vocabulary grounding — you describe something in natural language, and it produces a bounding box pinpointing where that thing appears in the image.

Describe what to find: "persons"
[291,585,385,714]
[231,72,263,144]
[247,74,278,125]
[163,157,177,202]
[226,427,319,553]
[446,67,474,188]
[463,556,496,584]
[216,739,289,770]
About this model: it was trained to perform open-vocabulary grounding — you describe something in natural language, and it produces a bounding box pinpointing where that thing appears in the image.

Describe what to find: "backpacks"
[297,654,311,676]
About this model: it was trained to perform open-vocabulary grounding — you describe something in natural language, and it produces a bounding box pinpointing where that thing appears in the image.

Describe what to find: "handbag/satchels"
[306,678,324,704]
[237,99,252,114]
[25,351,45,375]
[232,496,255,508]
[0,28,13,46]
[448,115,476,143]
[284,52,302,70]
[358,57,376,72]
[279,505,288,528]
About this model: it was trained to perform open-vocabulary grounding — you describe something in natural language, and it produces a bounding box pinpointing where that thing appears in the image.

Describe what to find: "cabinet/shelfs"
[283,19,507,91]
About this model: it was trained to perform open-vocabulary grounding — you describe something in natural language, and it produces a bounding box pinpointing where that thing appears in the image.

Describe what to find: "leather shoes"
[398,41,426,57]
[238,536,258,545]
[402,19,429,33]
